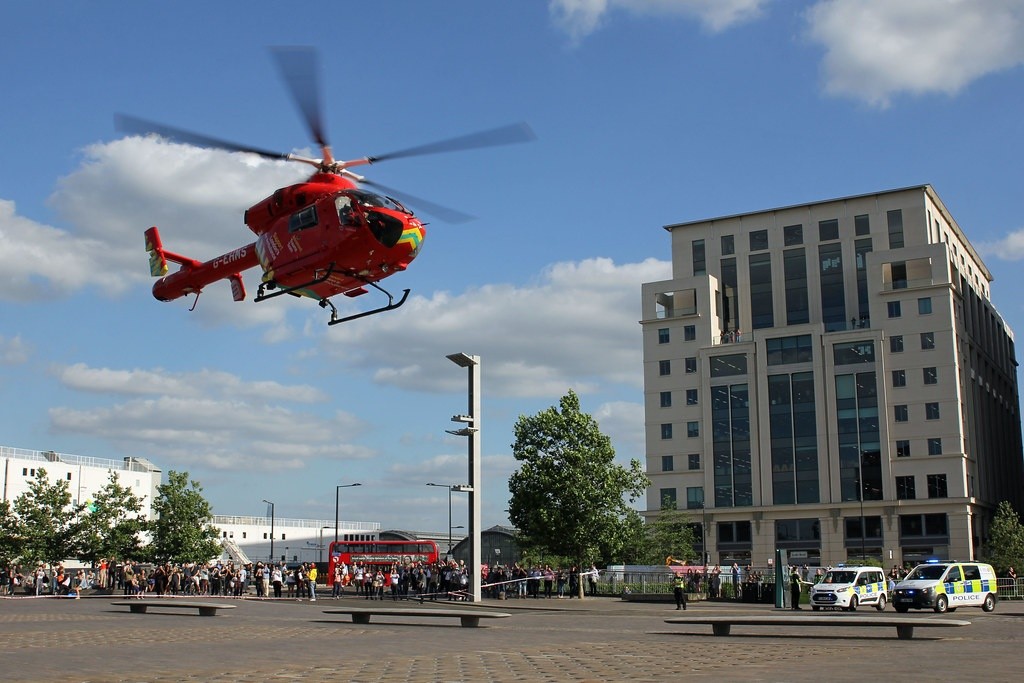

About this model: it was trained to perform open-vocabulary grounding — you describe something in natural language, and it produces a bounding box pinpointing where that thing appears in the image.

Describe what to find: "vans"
[893,558,999,613]
[809,562,888,611]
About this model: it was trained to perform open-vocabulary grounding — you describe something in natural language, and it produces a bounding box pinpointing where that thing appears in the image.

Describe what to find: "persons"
[672,571,686,610]
[588,563,598,594]
[730,562,764,598]
[0,556,318,601]
[390,559,579,604]
[888,565,909,596]
[686,563,721,597]
[404,556,414,568]
[332,560,385,600]
[790,566,802,610]
[787,562,833,583]
[860,314,866,327]
[344,197,370,223]
[736,330,741,342]
[1008,566,1016,590]
[851,317,856,329]
[720,330,734,344]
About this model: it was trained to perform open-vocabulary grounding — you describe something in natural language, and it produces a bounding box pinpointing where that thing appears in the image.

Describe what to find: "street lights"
[263,499,276,560]
[335,483,362,553]
[425,482,452,554]
[319,526,330,562]
[446,350,482,607]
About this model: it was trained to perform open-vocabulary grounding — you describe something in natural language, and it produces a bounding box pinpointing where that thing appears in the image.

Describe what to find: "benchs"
[110,601,239,617]
[323,608,511,628]
[664,617,972,640]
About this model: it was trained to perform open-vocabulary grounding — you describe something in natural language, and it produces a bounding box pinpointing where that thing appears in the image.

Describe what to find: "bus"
[326,539,440,587]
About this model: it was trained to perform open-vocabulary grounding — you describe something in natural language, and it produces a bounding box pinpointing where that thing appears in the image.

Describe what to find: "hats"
[310,563,316,568]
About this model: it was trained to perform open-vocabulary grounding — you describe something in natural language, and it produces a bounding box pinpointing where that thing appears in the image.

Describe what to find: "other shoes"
[676,607,681,610]
[683,608,686,610]
[310,598,316,601]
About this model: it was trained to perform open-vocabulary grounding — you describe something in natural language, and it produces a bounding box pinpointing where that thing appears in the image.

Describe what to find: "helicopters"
[111,43,536,331]
